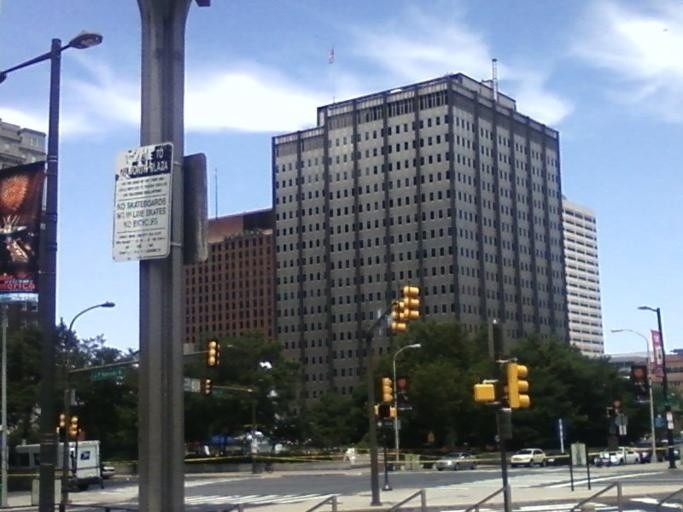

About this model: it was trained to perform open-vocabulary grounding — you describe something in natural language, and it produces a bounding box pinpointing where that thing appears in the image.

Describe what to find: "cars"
[386,453,433,470]
[436,451,478,471]
[547,451,571,464]
[595,446,640,465]
[638,440,679,461]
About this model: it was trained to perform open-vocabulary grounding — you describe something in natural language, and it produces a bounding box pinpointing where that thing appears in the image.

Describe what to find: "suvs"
[510,448,547,467]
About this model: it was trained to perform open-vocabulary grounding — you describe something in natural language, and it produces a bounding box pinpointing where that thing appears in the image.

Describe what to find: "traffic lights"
[202,376,213,395]
[506,362,531,409]
[381,377,394,402]
[208,338,221,367]
[612,399,623,417]
[391,284,420,331]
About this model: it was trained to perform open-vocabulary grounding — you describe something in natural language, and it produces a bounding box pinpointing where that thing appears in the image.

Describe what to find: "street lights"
[611,328,658,463]
[638,306,677,469]
[392,342,422,460]
